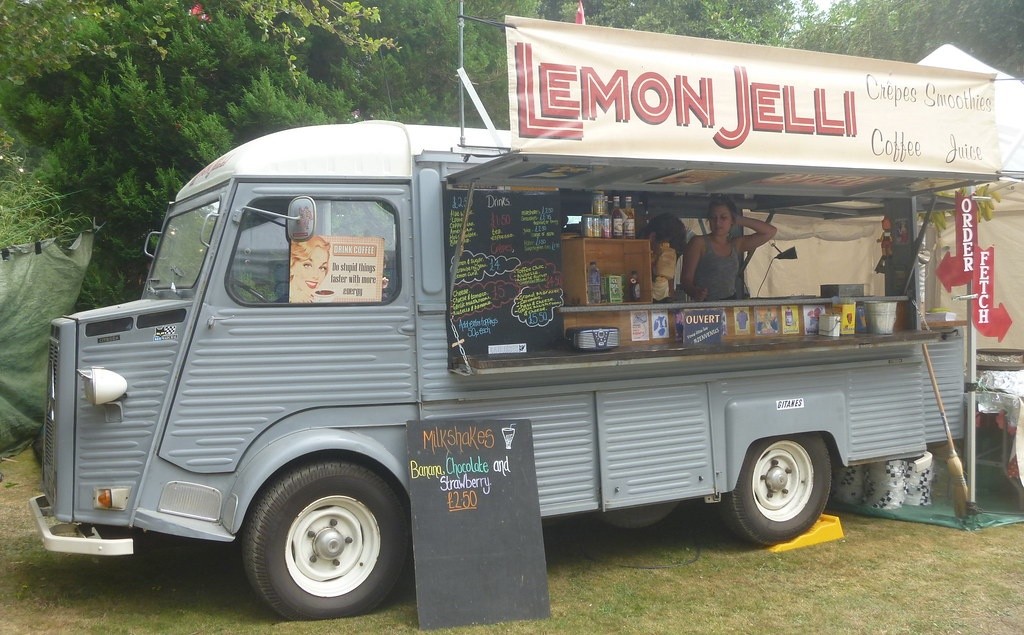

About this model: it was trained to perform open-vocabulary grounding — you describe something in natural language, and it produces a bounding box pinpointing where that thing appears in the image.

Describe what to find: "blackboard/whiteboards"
[443,188,566,356]
[406,418,552,629]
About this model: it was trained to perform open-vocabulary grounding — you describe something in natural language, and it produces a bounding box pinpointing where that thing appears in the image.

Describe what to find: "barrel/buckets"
[863,300,897,336]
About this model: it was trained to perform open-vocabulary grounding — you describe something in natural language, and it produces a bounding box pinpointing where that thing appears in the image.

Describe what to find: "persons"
[635,213,687,302]
[681,196,777,300]
[290,237,331,303]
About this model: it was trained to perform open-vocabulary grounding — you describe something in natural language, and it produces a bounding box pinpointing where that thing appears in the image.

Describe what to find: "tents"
[678,43,1024,528]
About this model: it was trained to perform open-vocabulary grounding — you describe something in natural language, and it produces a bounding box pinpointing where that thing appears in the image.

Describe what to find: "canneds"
[579,192,612,239]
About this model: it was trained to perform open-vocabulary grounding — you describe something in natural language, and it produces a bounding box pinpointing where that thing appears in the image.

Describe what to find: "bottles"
[587,261,601,303]
[856,303,868,334]
[785,307,793,327]
[630,270,641,302]
[580,190,646,240]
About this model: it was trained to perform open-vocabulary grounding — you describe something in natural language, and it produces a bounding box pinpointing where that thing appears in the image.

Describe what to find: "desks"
[976,391,1024,504]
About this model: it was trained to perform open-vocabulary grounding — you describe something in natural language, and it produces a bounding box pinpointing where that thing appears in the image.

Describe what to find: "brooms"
[921,342,971,519]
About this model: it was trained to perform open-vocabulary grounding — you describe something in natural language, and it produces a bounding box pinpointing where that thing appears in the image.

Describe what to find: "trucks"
[24,2,1024,622]
